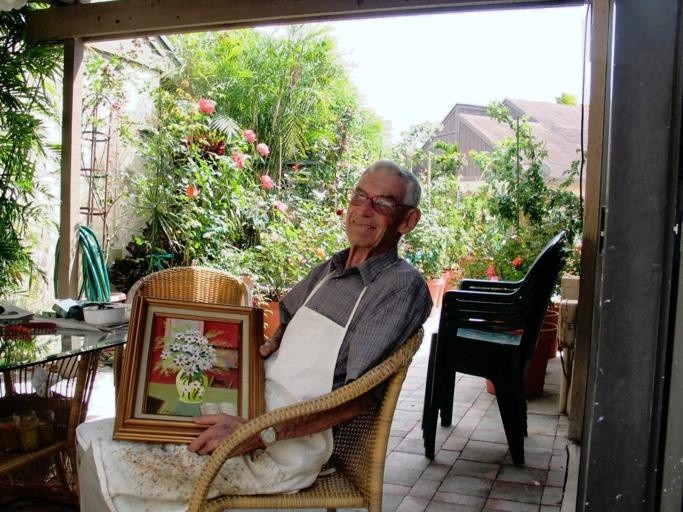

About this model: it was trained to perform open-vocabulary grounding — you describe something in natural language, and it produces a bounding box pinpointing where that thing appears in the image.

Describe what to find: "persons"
[74,161,435,511]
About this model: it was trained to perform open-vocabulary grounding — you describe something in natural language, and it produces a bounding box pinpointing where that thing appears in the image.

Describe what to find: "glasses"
[345,188,414,217]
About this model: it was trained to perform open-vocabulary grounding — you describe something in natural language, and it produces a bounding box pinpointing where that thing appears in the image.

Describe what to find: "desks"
[0,309,129,509]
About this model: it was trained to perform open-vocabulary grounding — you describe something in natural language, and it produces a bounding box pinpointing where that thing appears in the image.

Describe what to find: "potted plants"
[405,205,465,313]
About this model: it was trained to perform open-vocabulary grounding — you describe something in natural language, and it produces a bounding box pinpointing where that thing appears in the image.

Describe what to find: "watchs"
[259,426,278,449]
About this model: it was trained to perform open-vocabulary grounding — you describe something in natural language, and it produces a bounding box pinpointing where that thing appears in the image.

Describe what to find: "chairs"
[420,230,566,469]
